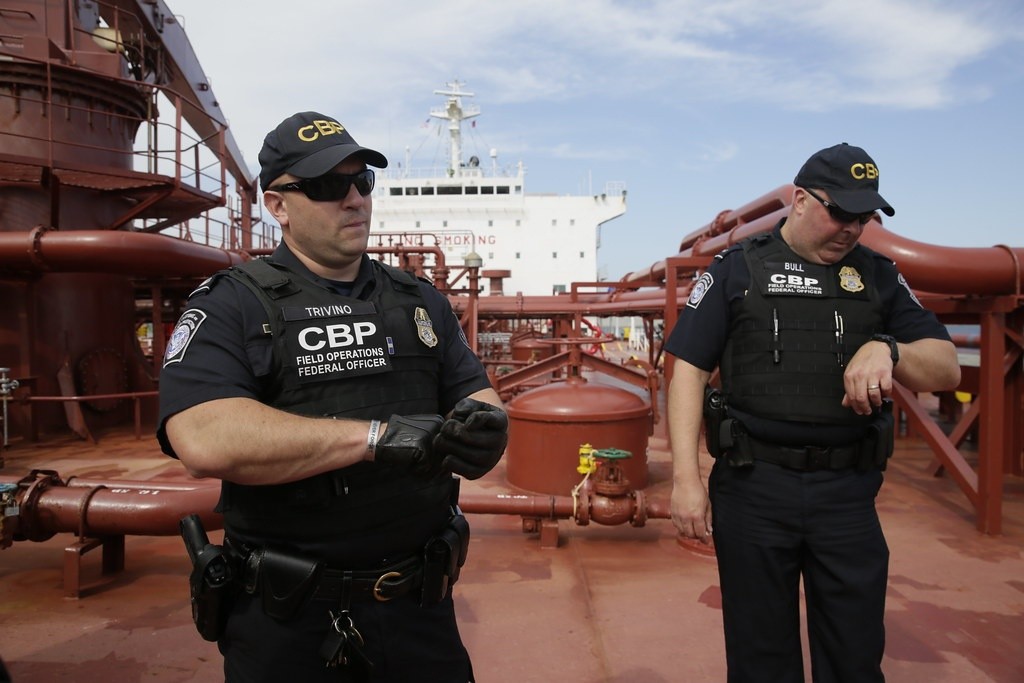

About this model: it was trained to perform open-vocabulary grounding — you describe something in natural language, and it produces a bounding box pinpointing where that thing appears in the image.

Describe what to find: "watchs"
[870,334,899,365]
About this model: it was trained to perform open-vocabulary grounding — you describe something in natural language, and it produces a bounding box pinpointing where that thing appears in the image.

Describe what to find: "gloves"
[374,414,446,484]
[432,398,508,480]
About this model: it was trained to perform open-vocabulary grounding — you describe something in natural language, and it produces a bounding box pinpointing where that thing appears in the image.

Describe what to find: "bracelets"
[364,420,381,462]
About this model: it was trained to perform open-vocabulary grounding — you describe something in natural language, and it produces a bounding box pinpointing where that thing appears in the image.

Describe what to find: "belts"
[750,438,859,471]
[317,566,424,602]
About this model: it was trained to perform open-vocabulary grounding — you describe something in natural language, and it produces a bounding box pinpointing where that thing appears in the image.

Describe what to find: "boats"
[365,78,629,336]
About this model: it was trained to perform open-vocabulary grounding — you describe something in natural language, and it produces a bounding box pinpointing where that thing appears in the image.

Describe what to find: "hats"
[794,142,895,217]
[258,111,388,192]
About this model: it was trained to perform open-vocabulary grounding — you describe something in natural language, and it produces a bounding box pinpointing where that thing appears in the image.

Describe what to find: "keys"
[318,627,364,668]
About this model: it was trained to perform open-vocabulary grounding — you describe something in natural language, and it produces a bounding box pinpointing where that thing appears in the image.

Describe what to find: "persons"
[662,144,961,683]
[153,111,509,683]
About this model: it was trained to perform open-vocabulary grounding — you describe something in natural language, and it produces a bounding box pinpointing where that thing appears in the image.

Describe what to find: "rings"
[868,385,880,389]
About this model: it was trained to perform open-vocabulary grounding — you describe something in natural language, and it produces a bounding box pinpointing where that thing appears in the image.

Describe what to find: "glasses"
[268,169,375,201]
[805,188,876,223]
